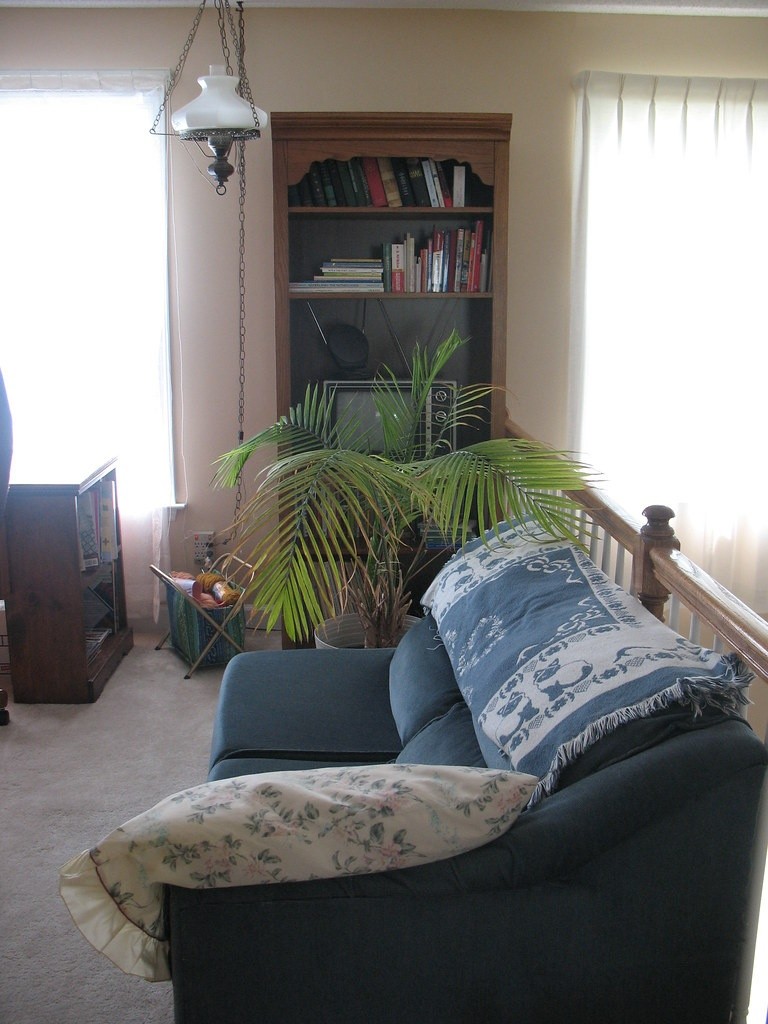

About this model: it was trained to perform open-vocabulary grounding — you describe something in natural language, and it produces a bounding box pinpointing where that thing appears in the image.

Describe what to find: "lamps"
[149,7,269,196]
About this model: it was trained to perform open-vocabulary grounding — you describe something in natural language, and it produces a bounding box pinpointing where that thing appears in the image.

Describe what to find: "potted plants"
[205,328,608,650]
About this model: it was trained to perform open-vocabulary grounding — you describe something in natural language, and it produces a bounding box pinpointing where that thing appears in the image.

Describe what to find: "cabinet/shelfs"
[264,108,512,650]
[0,458,133,705]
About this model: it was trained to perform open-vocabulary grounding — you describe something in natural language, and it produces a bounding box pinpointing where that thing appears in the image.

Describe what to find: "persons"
[0,375,11,728]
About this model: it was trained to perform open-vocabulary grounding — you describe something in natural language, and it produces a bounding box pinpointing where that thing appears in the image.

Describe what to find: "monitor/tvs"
[321,376,457,464]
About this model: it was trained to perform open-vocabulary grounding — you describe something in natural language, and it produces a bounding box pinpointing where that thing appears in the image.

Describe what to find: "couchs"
[139,513,768,1024]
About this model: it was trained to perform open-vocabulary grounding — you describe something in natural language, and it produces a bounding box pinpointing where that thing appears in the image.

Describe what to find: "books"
[288,156,465,207]
[78,480,118,572]
[288,219,489,293]
[85,628,110,665]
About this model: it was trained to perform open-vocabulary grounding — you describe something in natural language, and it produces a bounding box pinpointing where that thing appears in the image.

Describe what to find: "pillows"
[388,617,457,745]
[420,511,756,810]
[53,762,539,985]
[385,698,488,769]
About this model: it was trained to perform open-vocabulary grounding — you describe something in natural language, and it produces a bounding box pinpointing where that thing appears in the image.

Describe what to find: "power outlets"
[193,531,214,566]
